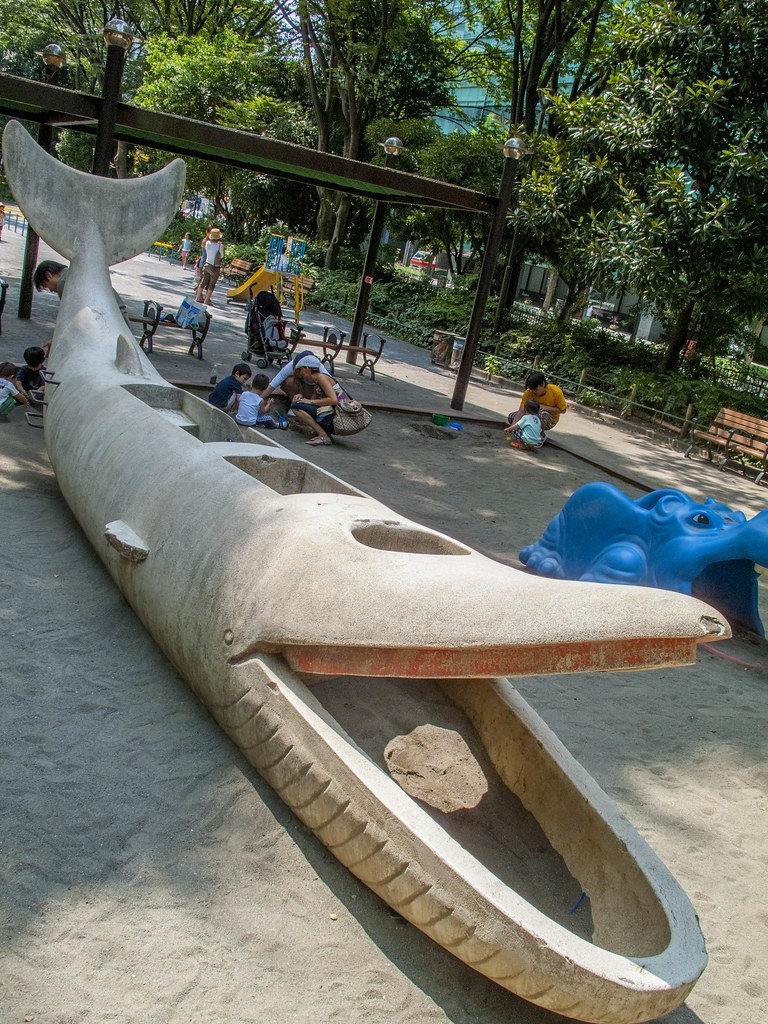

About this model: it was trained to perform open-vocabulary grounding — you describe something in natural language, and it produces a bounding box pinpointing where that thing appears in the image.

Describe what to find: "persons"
[235,374,289,429]
[505,398,542,449]
[176,232,195,269]
[15,346,47,397]
[208,363,251,413]
[32,260,130,329]
[0,362,30,422]
[197,228,224,305]
[508,371,567,448]
[194,227,213,298]
[0,204,5,241]
[259,352,331,416]
[291,355,352,446]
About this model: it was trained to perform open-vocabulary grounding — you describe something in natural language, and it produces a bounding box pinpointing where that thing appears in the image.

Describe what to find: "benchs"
[280,272,315,311]
[128,300,213,360]
[281,319,386,380]
[216,257,253,288]
[684,407,768,485]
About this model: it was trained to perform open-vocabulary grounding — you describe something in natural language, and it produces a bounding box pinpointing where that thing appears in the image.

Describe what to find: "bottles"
[209,365,217,384]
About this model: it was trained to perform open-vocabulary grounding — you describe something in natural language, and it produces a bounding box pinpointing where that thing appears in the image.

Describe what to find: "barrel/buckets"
[449,421,463,431]
[432,413,450,427]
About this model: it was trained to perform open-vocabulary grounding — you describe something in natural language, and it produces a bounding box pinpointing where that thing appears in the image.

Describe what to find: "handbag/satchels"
[334,398,373,436]
[214,242,223,267]
[174,294,206,330]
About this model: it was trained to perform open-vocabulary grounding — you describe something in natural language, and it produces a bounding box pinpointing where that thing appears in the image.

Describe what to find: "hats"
[159,310,177,323]
[209,228,223,240]
[295,355,321,369]
[293,350,314,363]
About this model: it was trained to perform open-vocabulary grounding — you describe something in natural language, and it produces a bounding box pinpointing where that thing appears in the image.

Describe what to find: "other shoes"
[277,421,289,428]
[195,297,204,303]
[183,266,186,269]
[228,410,237,416]
[535,435,548,448]
[305,436,334,446]
[203,299,213,305]
[510,440,525,449]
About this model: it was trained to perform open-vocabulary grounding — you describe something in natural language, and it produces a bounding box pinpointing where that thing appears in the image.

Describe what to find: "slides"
[227,263,277,303]
